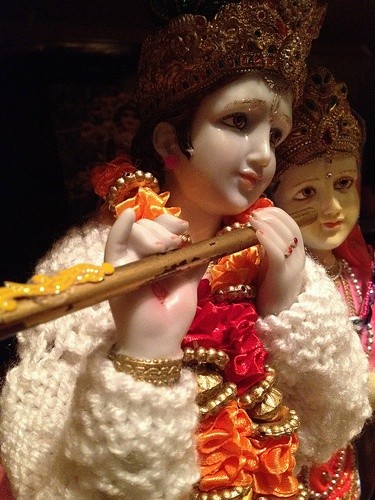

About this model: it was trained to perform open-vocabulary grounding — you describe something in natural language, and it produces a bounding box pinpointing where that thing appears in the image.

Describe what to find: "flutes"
[0,209,318,340]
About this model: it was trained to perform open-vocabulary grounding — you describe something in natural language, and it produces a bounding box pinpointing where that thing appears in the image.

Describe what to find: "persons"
[0,0,375,500]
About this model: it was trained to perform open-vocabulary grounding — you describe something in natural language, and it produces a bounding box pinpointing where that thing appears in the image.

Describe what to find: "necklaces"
[280,247,375,498]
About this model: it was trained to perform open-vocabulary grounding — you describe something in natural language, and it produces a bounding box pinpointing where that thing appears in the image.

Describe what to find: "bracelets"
[108,353,184,387]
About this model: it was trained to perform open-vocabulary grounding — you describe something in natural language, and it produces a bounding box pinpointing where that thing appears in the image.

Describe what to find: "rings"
[283,237,298,258]
